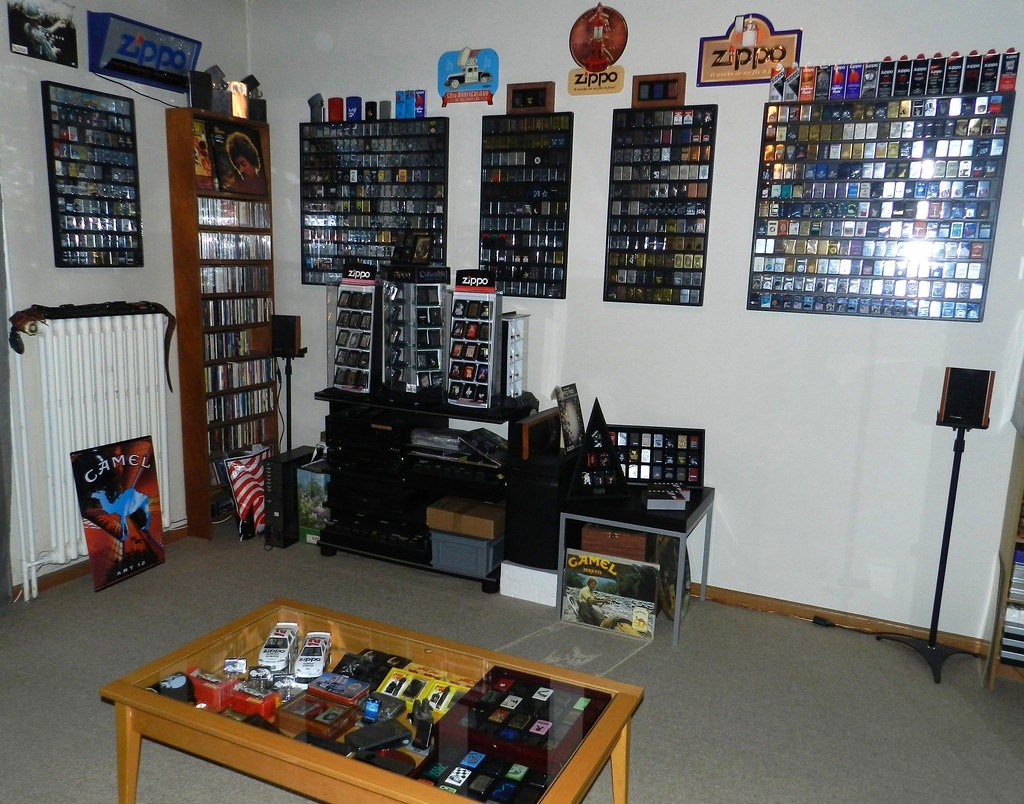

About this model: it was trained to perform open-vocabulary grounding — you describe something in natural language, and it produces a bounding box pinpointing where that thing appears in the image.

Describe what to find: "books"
[639,481,691,511]
[195,197,273,489]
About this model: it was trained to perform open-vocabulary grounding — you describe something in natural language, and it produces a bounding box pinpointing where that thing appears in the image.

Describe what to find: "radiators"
[7,313,169,600]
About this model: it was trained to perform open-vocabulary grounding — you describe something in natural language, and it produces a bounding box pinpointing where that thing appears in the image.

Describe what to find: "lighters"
[193,648,591,804]
[606,109,715,306]
[298,91,448,285]
[606,429,701,491]
[747,48,1022,321]
[479,116,569,300]
[332,284,494,406]
[47,85,143,268]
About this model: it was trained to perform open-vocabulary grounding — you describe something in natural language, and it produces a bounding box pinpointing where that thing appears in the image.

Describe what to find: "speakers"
[272,315,300,355]
[936,366,995,428]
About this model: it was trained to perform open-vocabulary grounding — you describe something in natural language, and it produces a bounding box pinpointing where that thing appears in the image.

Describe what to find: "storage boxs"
[297,458,331,545]
[264,445,323,548]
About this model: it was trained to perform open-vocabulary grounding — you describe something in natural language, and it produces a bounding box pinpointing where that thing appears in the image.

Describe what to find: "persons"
[578,578,610,626]
[23,0,68,62]
[226,132,266,194]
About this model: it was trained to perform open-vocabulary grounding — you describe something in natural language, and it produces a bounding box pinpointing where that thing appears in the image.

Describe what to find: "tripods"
[878,425,979,684]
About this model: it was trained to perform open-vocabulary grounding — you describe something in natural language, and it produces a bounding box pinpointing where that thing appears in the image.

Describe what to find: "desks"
[554,476,715,645]
[98,597,644,803]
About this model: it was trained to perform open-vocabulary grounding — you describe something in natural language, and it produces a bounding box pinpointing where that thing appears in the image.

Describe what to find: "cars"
[316,679,344,694]
[258,623,299,673]
[294,632,332,683]
[445,64,492,90]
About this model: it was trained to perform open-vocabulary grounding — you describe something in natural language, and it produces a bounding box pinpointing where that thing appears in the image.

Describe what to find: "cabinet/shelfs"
[164,109,281,539]
[314,386,540,595]
[984,432,1024,692]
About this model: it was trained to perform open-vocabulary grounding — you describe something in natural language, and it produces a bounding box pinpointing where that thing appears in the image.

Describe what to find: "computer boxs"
[264,445,324,548]
